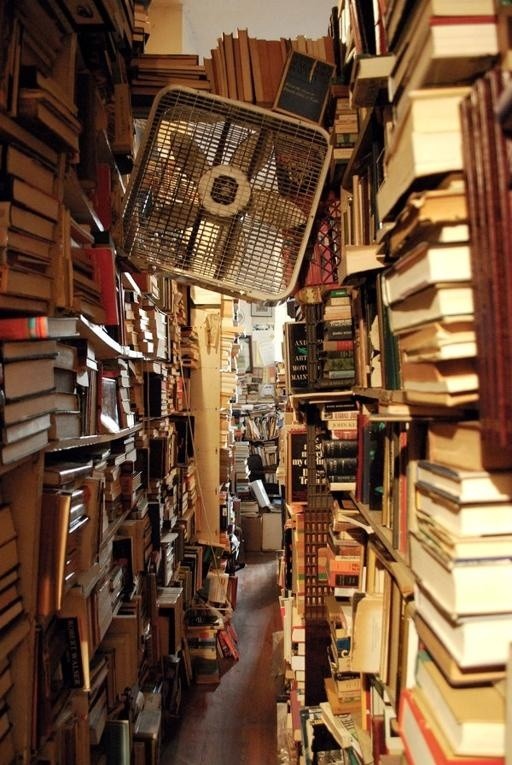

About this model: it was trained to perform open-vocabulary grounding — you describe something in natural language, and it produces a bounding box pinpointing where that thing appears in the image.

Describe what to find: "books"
[338,0,497,410]
[222,327,285,484]
[354,410,512,765]
[273,287,352,763]
[136,274,199,438]
[0,506,32,765]
[44,420,239,764]
[0,74,133,464]
[134,27,333,103]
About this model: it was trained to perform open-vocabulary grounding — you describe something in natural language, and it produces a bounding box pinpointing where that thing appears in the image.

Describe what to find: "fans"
[107,84,332,306]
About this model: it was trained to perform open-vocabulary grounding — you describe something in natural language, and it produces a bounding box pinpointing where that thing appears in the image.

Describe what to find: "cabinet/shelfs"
[278,4,512,764]
[0,3,236,759]
